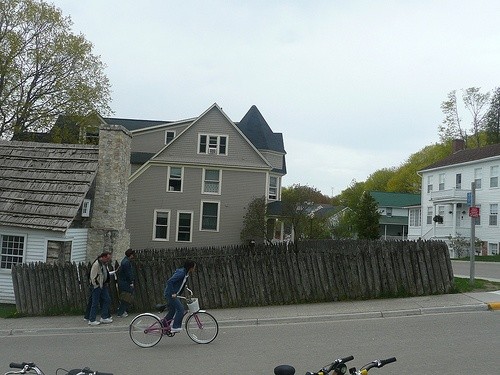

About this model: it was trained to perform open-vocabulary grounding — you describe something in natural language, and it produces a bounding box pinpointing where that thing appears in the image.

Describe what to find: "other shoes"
[171,327,182,332]
[84,319,88,321]
[100,318,113,323]
[118,312,128,317]
[88,321,100,325]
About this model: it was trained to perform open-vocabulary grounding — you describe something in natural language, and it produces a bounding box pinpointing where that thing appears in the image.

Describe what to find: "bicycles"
[128,288,219,348]
[4,361,114,375]
[274,355,397,375]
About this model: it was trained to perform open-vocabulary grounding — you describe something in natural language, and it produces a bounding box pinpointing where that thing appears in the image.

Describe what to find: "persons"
[82,251,120,327]
[161,259,198,332]
[116,248,136,318]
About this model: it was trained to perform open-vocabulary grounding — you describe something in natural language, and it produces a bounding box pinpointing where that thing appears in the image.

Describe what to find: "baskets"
[187,297,200,313]
